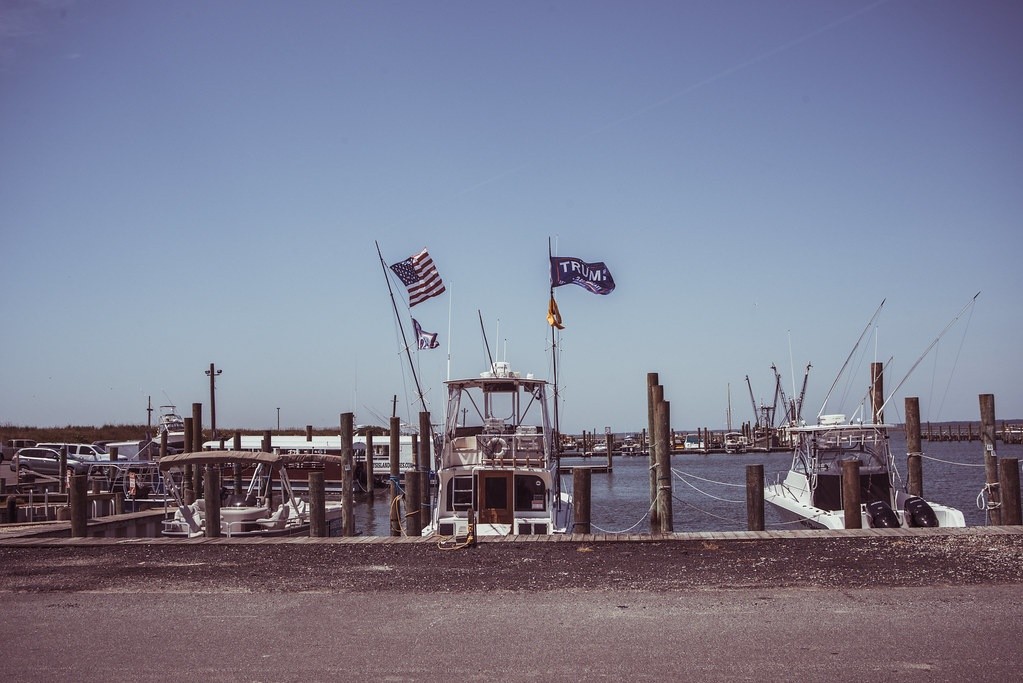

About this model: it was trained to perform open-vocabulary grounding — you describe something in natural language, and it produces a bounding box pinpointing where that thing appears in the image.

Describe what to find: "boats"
[764,291,981,528]
[685,434,706,448]
[158,451,357,537]
[420,279,573,536]
[621,430,641,456]
[745,362,813,447]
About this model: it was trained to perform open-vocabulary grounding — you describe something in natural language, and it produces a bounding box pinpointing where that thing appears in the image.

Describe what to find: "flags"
[413,318,439,350]
[551,256,616,295]
[391,247,446,307]
[547,298,566,330]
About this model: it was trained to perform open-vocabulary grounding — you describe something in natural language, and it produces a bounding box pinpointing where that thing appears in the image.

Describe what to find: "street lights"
[276,408,280,434]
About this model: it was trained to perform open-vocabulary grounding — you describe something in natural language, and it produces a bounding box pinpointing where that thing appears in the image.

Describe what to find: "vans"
[35,442,128,463]
[0,439,37,463]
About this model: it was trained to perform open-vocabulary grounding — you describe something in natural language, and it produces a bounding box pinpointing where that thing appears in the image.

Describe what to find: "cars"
[592,444,608,453]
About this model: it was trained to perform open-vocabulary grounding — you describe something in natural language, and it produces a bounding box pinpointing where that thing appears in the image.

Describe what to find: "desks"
[220,506,268,532]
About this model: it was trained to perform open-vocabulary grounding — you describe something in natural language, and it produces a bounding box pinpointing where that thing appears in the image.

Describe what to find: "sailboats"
[726,383,745,447]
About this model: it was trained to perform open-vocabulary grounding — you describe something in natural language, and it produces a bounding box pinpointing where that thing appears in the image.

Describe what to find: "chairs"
[256,504,290,531]
[193,499,207,518]
[272,497,306,527]
[176,506,200,532]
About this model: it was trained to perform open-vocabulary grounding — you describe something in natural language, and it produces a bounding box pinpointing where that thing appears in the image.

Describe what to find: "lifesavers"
[486,437,509,460]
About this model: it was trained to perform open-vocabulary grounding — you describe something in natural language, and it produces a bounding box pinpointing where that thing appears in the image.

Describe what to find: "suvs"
[10,447,95,478]
